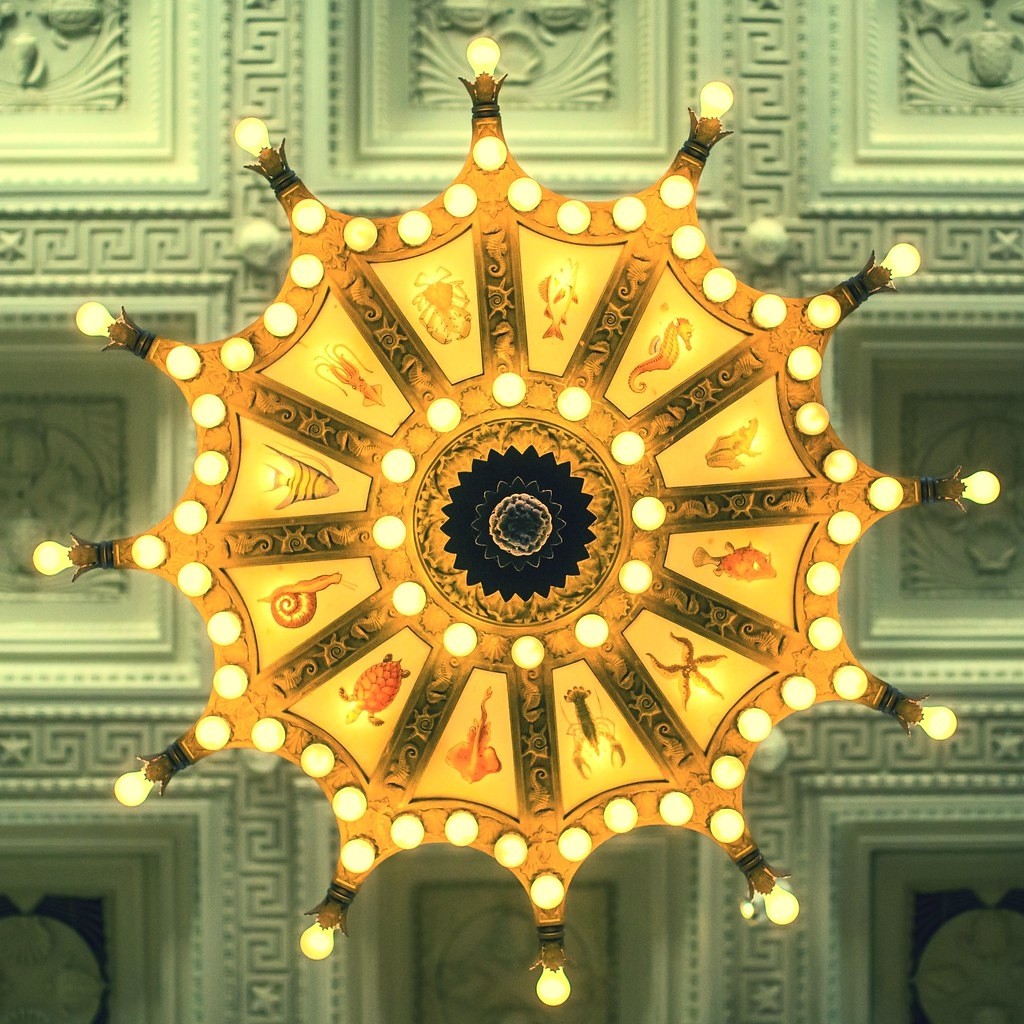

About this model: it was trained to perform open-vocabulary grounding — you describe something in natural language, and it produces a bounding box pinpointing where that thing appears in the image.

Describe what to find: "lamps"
[34,35,1000,1007]
